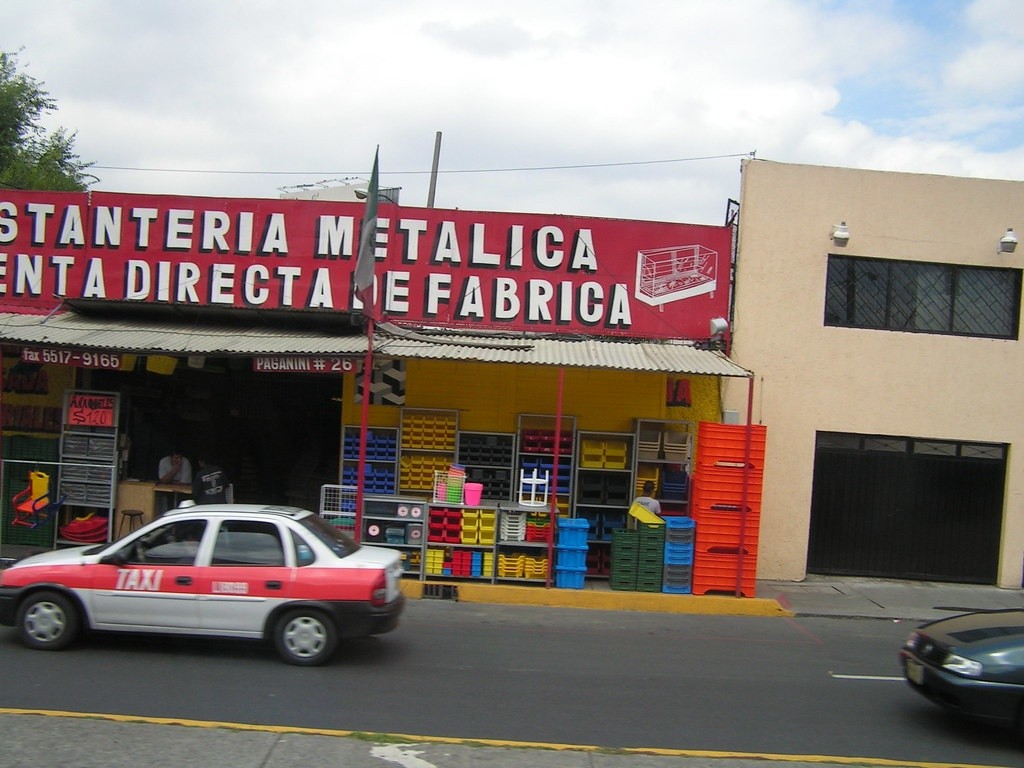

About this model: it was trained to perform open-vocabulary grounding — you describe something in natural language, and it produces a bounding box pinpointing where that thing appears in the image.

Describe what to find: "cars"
[0,499,403,669]
[898,604,1024,730]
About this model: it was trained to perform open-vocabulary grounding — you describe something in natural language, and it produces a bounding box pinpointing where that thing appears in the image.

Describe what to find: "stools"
[199,524,229,546]
[118,510,144,540]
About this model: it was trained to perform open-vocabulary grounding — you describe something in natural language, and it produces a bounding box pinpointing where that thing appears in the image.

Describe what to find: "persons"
[155,448,192,514]
[191,456,229,506]
[632,480,661,531]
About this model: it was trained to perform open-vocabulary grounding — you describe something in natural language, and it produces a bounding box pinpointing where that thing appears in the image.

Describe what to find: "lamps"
[997,228,1018,255]
[829,221,850,248]
[710,317,728,342]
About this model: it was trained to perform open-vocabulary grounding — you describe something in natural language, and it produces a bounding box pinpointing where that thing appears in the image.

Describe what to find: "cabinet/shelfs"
[54,388,121,551]
[320,407,696,589]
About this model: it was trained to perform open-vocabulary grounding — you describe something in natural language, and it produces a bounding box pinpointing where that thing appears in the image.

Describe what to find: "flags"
[354,159,379,318]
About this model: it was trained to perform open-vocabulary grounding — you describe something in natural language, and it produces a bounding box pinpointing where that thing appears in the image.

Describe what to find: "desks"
[154,483,193,535]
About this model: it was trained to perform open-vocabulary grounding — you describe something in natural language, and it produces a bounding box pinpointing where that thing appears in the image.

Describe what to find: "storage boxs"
[340,412,767,598]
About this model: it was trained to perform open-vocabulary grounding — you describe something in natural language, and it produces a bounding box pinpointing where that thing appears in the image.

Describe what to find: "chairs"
[11,470,70,529]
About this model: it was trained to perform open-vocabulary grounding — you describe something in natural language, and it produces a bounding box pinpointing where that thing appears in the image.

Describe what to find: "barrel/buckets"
[465,483,483,505]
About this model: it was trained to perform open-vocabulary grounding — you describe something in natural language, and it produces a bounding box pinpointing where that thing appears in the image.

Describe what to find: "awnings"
[0,311,370,359]
[373,335,754,597]
